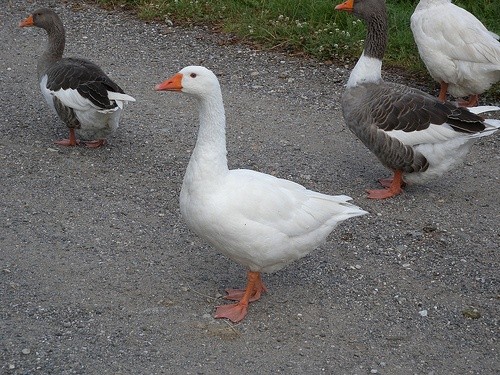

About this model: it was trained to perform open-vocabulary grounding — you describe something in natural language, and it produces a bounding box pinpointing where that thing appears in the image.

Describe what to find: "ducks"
[18,5,137,153]
[151,63,372,331]
[331,0,500,201]
